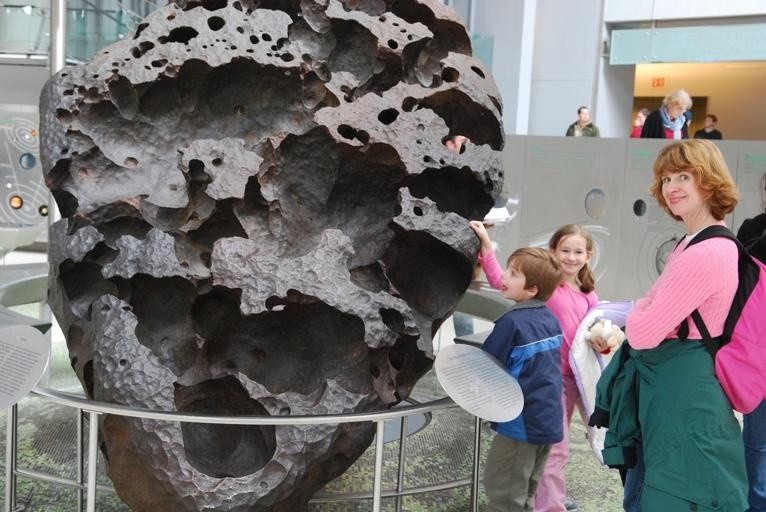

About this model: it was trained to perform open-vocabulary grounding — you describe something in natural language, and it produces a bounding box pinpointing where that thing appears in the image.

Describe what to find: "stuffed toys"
[584,316,623,353]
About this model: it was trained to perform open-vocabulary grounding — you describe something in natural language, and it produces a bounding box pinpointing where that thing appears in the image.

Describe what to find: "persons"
[628,107,650,137]
[564,106,599,137]
[625,138,751,512]
[470,218,612,512]
[694,114,721,139]
[640,88,694,141]
[735,173,766,511]
[441,132,497,338]
[683,110,693,127]
[479,245,565,512]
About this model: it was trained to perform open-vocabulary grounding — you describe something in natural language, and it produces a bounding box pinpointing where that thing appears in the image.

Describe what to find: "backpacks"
[672,225,766,415]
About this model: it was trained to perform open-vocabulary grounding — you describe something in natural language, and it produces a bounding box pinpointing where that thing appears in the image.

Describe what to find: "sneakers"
[564,496,578,512]
